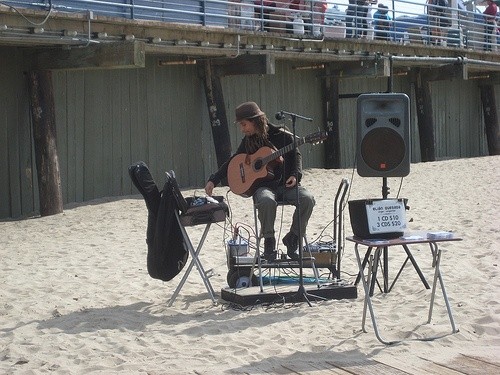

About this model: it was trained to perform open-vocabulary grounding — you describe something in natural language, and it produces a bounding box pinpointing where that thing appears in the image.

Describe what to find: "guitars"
[227,129,329,197]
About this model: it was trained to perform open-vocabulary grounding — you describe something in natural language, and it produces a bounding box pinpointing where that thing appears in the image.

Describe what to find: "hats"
[235,102,265,122]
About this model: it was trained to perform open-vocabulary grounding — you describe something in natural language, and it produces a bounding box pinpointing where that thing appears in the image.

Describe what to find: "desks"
[345,230,464,345]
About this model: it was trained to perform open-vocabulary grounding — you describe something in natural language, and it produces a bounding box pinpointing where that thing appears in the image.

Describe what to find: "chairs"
[164,169,230,306]
[247,195,321,293]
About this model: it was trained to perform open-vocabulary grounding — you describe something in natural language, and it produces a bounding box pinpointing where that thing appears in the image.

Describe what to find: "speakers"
[356,93,410,177]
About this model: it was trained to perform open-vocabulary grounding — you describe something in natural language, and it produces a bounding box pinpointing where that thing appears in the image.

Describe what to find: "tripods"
[279,112,328,308]
[355,177,430,294]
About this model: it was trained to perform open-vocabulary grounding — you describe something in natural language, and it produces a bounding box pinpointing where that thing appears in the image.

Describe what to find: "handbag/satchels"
[348,198,408,239]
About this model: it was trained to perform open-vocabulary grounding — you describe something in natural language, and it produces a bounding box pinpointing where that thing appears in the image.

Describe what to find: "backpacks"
[143,181,189,281]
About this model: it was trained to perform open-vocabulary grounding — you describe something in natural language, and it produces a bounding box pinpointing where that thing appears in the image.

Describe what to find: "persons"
[427,0,451,46]
[255,1,326,34]
[345,0,391,40]
[205,102,316,260]
[483,0,497,50]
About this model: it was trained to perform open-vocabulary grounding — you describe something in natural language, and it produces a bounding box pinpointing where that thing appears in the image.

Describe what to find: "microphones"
[276,111,285,120]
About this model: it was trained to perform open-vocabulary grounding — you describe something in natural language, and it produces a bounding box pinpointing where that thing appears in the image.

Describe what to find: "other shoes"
[264,237,277,260]
[282,232,300,260]
